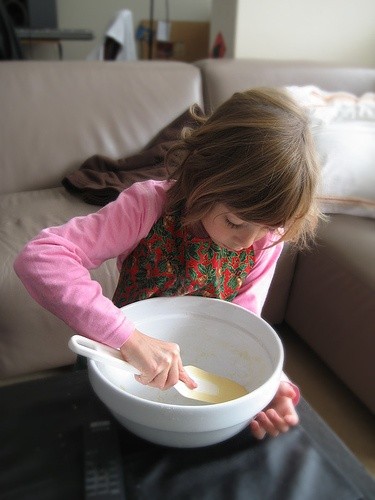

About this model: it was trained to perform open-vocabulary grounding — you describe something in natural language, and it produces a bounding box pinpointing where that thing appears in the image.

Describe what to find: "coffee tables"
[1,365,375,500]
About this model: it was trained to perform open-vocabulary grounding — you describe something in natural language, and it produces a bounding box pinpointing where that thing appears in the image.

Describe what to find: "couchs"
[1,53,375,411]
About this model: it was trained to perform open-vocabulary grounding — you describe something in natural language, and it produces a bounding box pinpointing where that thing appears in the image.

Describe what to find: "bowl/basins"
[87,295,284,448]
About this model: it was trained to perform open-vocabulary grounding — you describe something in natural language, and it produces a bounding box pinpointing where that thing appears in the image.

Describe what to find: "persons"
[12,86,323,437]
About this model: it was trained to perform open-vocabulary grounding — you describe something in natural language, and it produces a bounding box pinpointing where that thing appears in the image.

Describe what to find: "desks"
[15,27,95,59]
